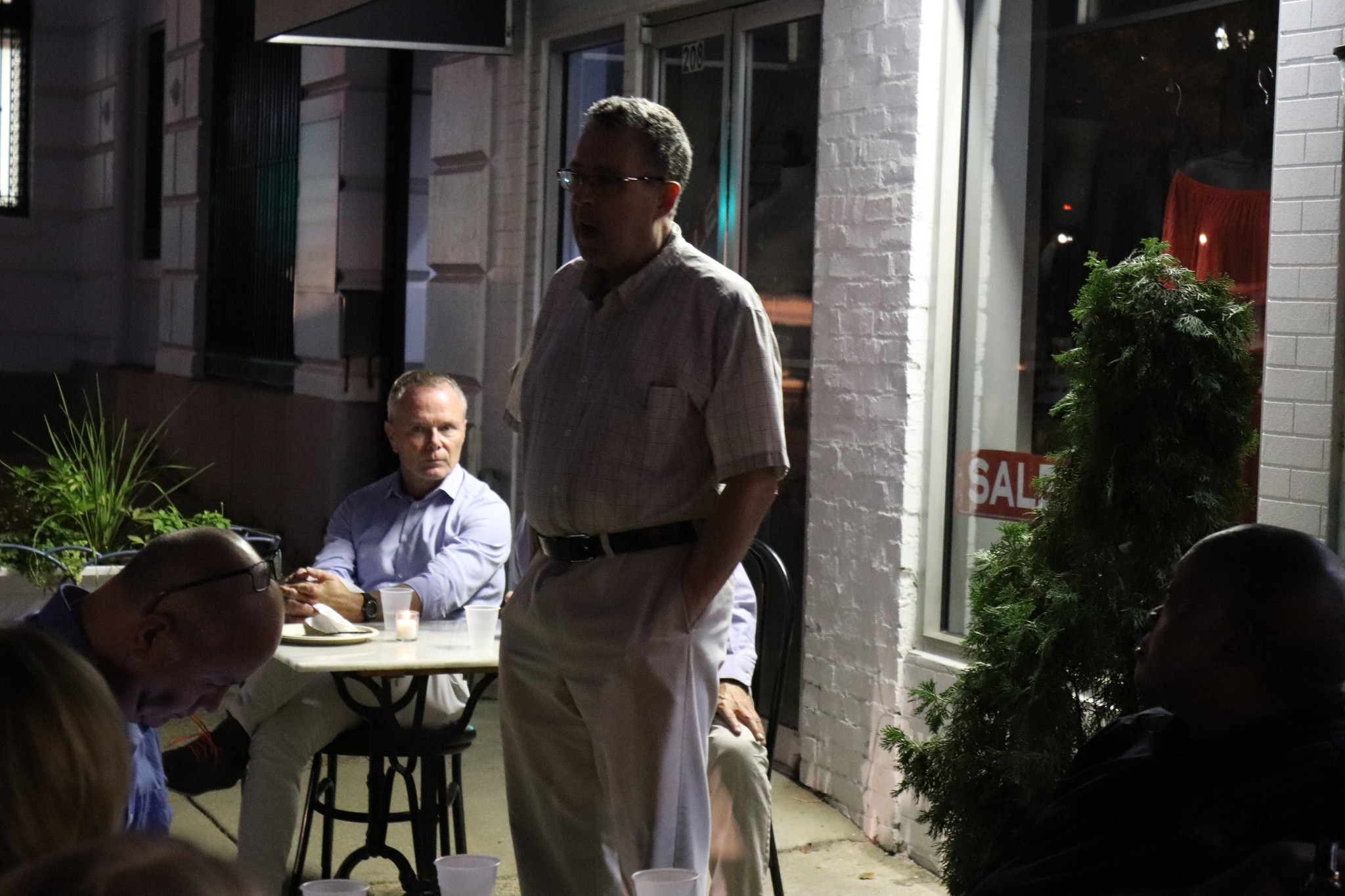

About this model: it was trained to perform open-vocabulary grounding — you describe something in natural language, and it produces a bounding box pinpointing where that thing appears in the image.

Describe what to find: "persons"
[1048,522,1345,896]
[0,524,286,896]
[160,367,514,896]
[505,512,773,896]
[498,94,793,896]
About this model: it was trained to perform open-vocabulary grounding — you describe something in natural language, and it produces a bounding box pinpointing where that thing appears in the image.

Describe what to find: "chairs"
[725,540,799,896]
[294,674,480,895]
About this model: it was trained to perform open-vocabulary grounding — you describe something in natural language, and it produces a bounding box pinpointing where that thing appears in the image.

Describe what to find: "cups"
[631,867,700,896]
[299,879,372,896]
[380,587,414,631]
[433,852,501,896]
[396,610,421,642]
[464,605,501,646]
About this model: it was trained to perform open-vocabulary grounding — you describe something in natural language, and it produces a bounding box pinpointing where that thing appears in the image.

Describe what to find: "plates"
[280,623,379,645]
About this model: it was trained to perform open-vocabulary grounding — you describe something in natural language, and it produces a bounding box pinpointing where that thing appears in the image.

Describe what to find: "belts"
[535,517,700,563]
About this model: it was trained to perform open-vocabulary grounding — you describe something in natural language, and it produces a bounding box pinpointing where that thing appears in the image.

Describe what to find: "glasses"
[556,159,687,197]
[147,532,285,609]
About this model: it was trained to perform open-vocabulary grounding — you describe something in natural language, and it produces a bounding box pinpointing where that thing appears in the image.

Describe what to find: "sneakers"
[160,712,252,796]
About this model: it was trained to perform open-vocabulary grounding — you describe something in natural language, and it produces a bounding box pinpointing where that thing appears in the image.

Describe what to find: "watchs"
[356,592,379,624]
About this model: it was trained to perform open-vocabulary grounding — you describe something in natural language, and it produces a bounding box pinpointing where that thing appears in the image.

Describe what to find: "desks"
[270,617,503,896]
